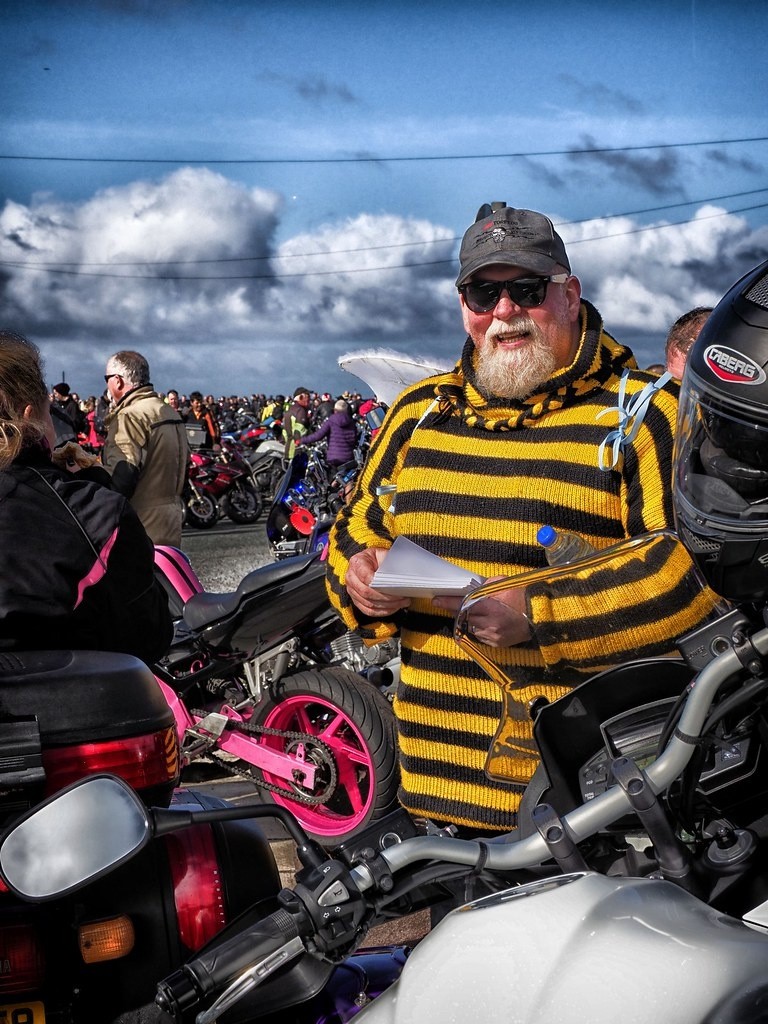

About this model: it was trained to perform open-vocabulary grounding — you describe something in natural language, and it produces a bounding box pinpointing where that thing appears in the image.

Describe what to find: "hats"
[454,201,571,287]
[54,383,71,396]
[293,387,314,397]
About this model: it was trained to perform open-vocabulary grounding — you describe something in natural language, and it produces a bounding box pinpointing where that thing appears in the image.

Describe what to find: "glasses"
[457,271,568,314]
[104,373,123,384]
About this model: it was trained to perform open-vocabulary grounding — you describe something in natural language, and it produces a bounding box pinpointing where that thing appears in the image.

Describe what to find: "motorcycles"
[0,425,768,1024]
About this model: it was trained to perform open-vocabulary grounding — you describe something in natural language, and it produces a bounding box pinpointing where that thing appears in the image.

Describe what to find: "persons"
[282,387,314,460]
[0,331,175,664]
[185,392,222,450]
[66,351,188,549]
[325,207,721,930]
[159,390,362,422]
[665,306,716,379]
[295,400,358,485]
[49,383,114,465]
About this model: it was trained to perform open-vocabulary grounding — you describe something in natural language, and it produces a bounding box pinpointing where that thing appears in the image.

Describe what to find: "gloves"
[295,438,300,446]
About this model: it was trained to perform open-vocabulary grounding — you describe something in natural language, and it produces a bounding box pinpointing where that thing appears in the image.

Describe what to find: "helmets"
[670,255,768,602]
[321,393,331,402]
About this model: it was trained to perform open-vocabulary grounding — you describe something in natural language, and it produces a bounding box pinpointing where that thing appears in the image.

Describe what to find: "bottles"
[537,526,598,566]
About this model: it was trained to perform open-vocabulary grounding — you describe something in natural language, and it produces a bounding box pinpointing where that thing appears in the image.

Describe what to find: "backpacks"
[272,403,285,420]
